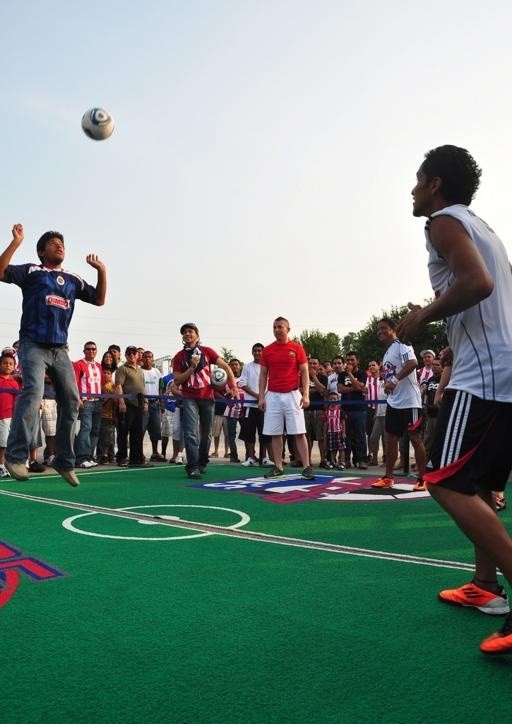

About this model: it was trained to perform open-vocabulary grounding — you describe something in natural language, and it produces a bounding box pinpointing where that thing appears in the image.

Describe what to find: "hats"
[420,349,435,357]
[181,323,198,335]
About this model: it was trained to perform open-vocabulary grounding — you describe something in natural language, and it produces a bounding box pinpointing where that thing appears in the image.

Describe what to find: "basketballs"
[211,369,227,386]
[81,107,116,139]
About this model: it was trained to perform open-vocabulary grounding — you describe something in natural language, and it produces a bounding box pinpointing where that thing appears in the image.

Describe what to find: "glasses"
[126,351,138,356]
[84,347,98,351]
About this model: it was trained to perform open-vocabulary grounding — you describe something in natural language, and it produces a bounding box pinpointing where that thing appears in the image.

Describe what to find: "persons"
[291,357,327,468]
[214,359,260,463]
[434,345,454,408]
[40,371,58,466]
[259,317,317,479]
[100,352,121,385]
[327,356,346,467]
[362,361,383,462]
[115,346,154,468]
[371,319,426,491]
[366,366,389,467]
[140,351,168,462]
[338,351,368,470]
[173,323,239,480]
[415,358,443,478]
[319,363,327,377]
[0,353,22,477]
[323,361,334,377]
[2,347,22,377]
[412,350,436,468]
[322,392,348,470]
[29,404,46,473]
[136,348,145,367]
[240,343,275,466]
[98,368,117,464]
[14,340,20,354]
[396,144,512,653]
[162,372,180,463]
[209,384,231,458]
[1,224,106,487]
[108,345,124,369]
[73,341,109,469]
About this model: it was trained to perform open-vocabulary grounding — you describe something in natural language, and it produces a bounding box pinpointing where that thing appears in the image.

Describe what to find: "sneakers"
[480,624,512,653]
[188,467,203,479]
[264,466,284,478]
[4,460,30,482]
[302,466,316,480]
[261,459,274,466]
[414,478,428,491]
[79,461,92,467]
[439,584,510,615]
[58,469,81,487]
[242,457,259,467]
[371,478,395,488]
[1,464,9,478]
